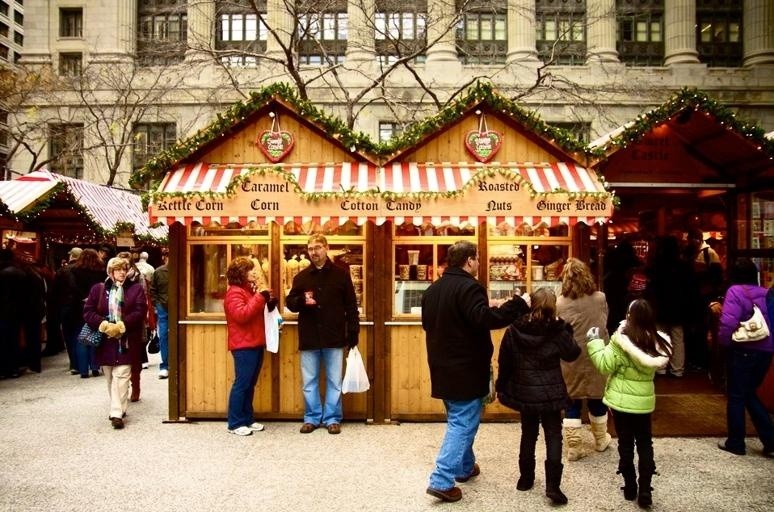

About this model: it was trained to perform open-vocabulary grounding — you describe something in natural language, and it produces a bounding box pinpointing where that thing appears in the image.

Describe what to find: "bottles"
[489,256,520,283]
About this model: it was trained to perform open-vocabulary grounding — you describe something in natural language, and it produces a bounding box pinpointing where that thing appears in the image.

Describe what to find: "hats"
[67,248,82,257]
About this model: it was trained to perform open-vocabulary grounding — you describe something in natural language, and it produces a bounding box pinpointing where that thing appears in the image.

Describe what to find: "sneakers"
[226,422,264,436]
[717,439,728,449]
[158,368,168,377]
[456,462,479,482]
[427,484,462,500]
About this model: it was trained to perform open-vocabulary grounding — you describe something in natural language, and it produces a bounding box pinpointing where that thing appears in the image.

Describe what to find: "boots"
[517,456,535,491]
[638,459,660,509]
[618,460,637,499]
[544,459,568,504]
[131,372,140,402]
[562,418,587,461]
[588,411,611,451]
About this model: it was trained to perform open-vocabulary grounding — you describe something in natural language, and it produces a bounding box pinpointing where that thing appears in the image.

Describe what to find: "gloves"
[585,327,601,341]
[99,320,126,339]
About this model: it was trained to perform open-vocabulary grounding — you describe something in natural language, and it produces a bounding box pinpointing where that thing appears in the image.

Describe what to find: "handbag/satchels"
[731,304,770,344]
[148,335,160,353]
[77,322,102,348]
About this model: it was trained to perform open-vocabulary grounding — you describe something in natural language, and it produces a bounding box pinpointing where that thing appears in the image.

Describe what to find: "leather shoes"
[327,424,341,433]
[300,423,315,433]
[112,418,123,427]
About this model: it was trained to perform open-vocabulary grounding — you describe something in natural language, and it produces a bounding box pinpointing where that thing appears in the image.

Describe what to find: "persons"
[224,257,277,437]
[286,235,360,433]
[421,240,532,501]
[496,288,582,503]
[558,212,774,508]
[0,246,169,429]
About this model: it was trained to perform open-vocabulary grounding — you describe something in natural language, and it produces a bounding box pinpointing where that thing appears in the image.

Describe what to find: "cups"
[349,265,364,308]
[532,266,544,281]
[399,251,429,280]
